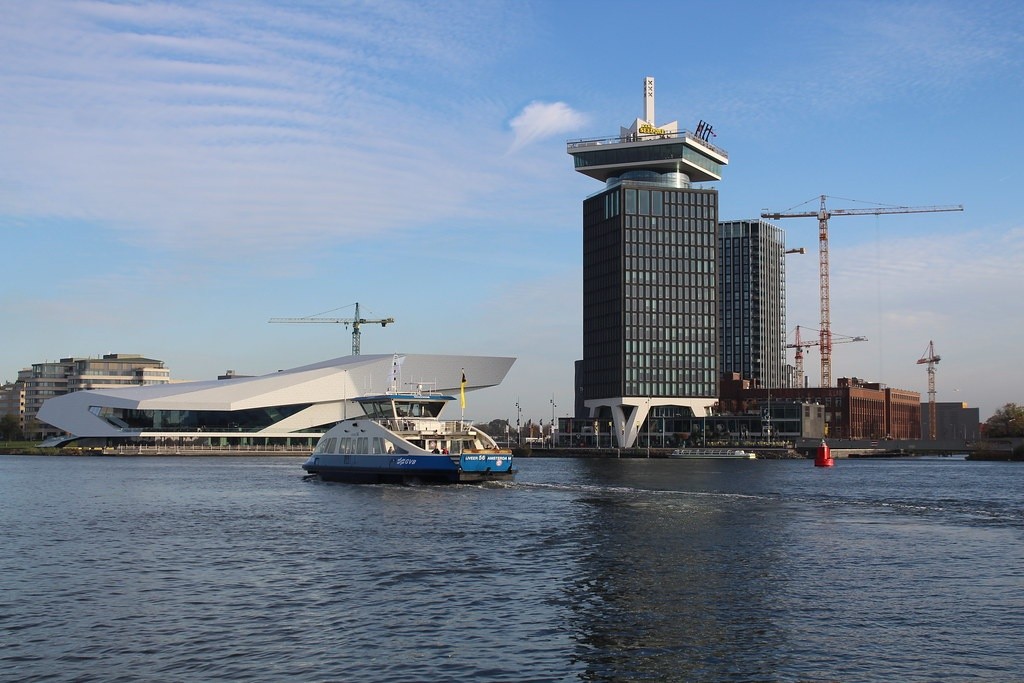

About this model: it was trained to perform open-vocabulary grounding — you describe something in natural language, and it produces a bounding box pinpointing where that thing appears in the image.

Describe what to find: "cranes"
[914,339,942,440]
[265,302,396,356]
[759,194,965,387]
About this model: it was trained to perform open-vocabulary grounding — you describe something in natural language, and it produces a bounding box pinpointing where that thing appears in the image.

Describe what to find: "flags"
[460,370,468,409]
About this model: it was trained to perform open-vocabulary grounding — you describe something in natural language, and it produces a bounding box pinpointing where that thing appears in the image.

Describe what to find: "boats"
[666,449,755,459]
[300,352,518,485]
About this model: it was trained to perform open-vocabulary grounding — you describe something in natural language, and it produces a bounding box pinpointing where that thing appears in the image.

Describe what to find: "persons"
[388,444,498,455]
